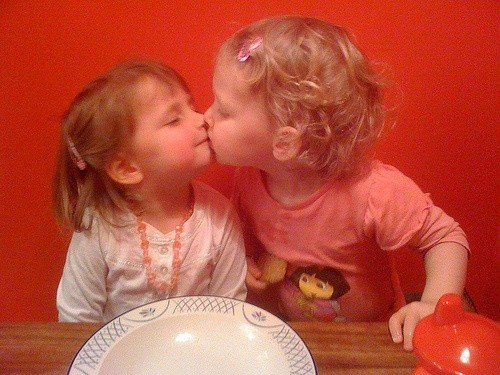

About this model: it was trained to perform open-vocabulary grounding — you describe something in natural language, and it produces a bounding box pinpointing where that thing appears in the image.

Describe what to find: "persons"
[53,56,248,323]
[203,15,471,352]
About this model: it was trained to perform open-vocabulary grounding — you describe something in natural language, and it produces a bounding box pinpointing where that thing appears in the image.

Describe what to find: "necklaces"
[134,185,195,300]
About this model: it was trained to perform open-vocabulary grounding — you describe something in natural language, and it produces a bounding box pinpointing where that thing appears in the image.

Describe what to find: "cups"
[409,294,500,374]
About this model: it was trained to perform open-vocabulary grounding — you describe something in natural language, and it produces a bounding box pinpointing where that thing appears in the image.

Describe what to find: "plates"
[65,295,318,375]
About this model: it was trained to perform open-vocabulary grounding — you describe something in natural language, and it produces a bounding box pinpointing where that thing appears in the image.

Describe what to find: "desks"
[0,321,418,375]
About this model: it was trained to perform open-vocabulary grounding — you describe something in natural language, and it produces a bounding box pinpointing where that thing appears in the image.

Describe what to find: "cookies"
[254,251,288,285]
